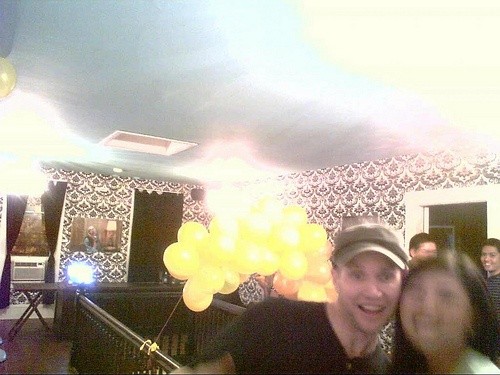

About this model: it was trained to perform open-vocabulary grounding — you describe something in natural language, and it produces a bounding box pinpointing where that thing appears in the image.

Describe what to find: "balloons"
[163,195,338,312]
[0,57,16,99]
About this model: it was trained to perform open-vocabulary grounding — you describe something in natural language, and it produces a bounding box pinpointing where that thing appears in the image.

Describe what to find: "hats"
[329,224,408,270]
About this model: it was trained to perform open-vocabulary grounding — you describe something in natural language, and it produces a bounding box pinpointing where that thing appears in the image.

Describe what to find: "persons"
[170,224,409,375]
[84,226,102,254]
[410,232,438,258]
[480,238,499,310]
[394,248,500,375]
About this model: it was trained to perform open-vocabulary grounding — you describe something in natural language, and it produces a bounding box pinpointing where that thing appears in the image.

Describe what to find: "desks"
[8,282,183,339]
